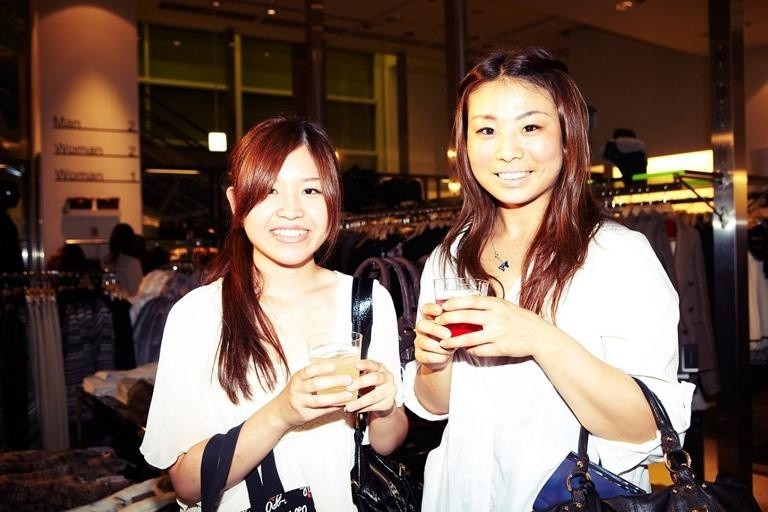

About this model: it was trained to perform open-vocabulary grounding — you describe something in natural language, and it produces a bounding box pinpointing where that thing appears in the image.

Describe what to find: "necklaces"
[489,237,510,272]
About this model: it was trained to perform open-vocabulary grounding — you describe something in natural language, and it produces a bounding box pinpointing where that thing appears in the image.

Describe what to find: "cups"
[304,330,364,407]
[432,277,491,337]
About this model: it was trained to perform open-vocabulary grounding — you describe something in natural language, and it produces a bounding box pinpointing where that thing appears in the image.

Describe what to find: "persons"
[138,116,410,511]
[599,128,647,190]
[54,223,172,279]
[397,47,694,511]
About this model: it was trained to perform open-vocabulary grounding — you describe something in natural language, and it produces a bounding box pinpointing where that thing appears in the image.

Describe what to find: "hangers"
[350,195,459,241]
[604,185,710,227]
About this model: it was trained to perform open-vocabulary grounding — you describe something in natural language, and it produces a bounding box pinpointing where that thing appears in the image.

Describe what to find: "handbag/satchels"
[352,446,425,511]
[201,421,316,511]
[533,452,645,511]
[540,376,765,511]
